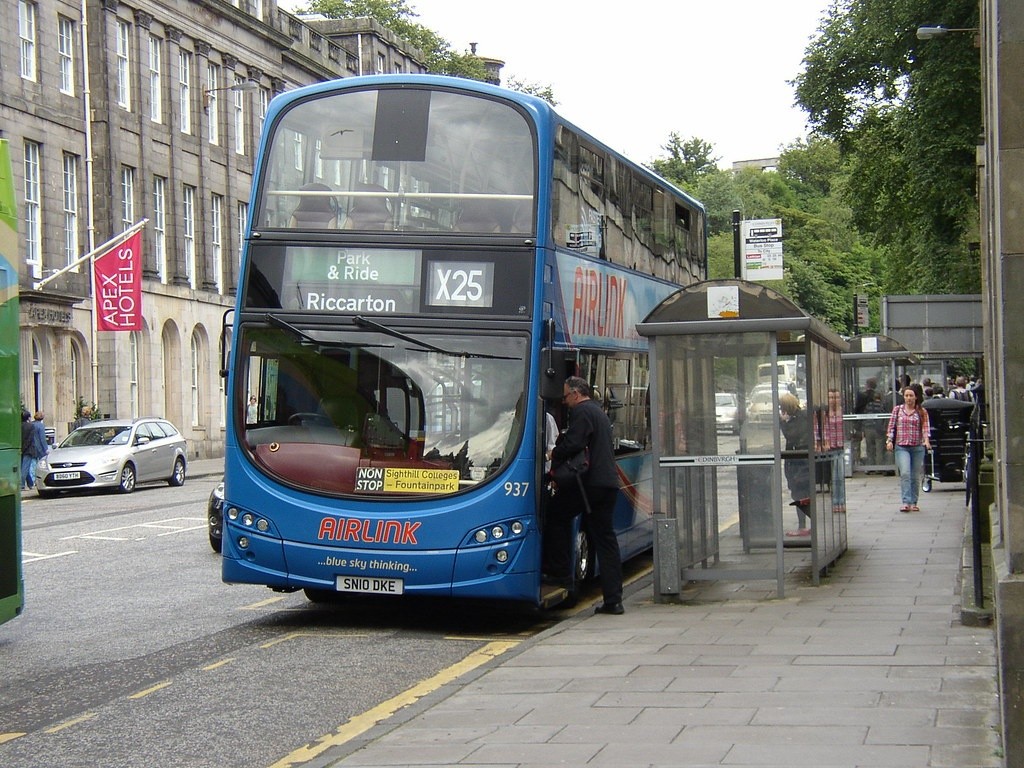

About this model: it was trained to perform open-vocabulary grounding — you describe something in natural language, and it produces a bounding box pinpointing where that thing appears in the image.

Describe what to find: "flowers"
[68,396,101,419]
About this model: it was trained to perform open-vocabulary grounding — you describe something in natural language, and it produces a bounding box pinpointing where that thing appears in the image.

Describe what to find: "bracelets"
[545,454,549,460]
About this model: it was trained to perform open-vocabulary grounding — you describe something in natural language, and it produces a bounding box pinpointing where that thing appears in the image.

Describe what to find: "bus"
[756,335,808,389]
[219,74,706,620]
[0,139,26,626]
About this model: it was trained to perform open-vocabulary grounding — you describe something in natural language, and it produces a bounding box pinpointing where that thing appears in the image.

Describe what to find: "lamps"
[202,82,258,107]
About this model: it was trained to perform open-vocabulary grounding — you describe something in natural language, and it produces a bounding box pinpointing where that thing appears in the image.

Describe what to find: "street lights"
[853,282,876,336]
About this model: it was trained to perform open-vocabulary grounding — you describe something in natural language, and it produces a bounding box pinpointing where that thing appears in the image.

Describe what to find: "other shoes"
[911,505,918,511]
[786,529,808,535]
[900,504,910,510]
[832,505,845,512]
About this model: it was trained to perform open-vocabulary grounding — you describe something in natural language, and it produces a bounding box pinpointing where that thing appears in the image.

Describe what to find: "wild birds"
[330,129,354,136]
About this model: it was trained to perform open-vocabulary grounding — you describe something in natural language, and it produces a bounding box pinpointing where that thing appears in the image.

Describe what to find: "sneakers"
[596,601,624,614]
[545,576,575,591]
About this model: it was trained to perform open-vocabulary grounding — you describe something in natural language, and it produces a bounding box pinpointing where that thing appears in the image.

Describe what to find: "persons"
[247,395,257,424]
[545,376,625,614]
[886,386,932,511]
[29,412,48,484]
[779,393,811,536]
[21,411,36,490]
[923,375,974,402]
[75,406,91,428]
[854,375,911,476]
[824,389,845,512]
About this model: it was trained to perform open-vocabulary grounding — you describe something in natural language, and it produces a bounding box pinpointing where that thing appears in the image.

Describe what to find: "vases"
[68,421,100,434]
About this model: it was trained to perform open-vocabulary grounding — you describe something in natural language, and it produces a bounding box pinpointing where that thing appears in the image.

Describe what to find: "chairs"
[288,183,453,231]
[451,199,533,233]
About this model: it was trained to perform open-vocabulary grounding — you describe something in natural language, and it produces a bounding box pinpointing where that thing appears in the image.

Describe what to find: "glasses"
[562,392,572,400]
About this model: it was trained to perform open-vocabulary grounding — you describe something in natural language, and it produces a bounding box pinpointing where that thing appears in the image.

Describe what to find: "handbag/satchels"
[548,429,590,494]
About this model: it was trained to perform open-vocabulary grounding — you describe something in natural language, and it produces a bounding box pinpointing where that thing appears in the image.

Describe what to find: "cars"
[715,381,807,436]
[34,416,186,499]
[208,477,224,552]
